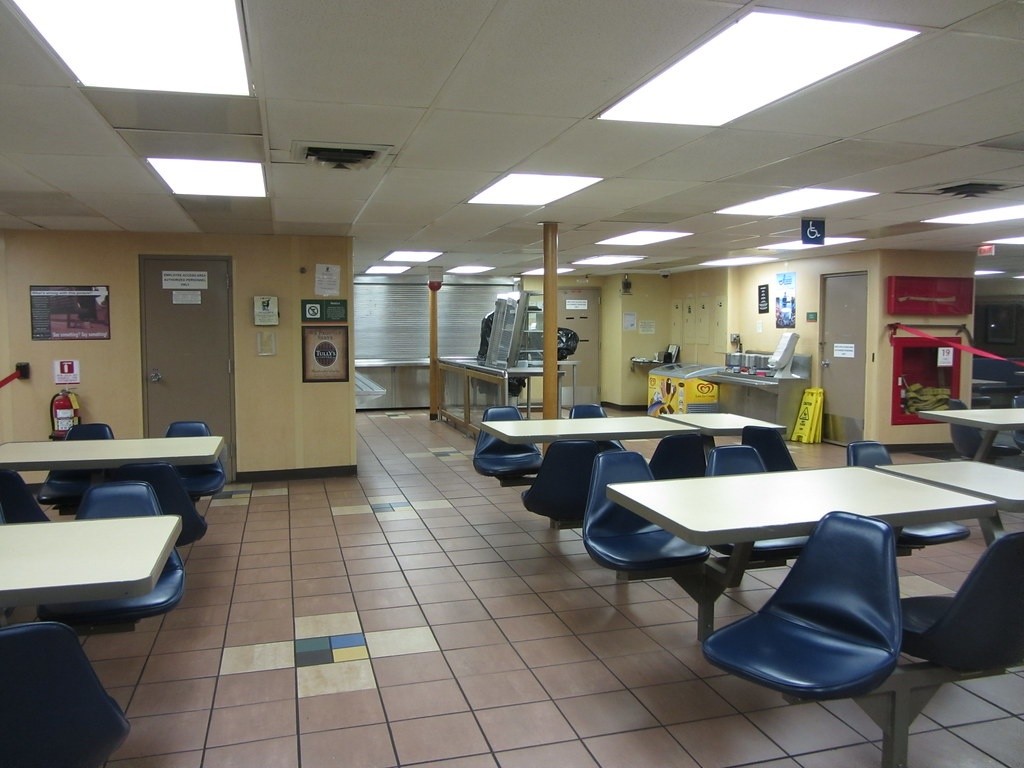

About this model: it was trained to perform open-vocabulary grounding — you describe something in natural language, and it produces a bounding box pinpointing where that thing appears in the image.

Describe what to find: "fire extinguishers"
[49,386,81,441]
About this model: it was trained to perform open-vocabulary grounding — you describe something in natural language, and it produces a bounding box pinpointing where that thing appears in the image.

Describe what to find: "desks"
[917,408,1024,463]
[605,465,996,588]
[873,460,1024,513]
[0,514,183,607]
[972,379,1007,389]
[658,413,787,437]
[0,436,224,471]
[479,416,702,444]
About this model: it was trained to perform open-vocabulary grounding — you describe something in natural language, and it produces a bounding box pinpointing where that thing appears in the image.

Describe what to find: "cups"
[732,367,740,373]
[748,369,756,374]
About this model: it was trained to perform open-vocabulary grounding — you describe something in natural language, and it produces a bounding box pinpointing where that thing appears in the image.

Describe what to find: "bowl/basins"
[756,371,766,377]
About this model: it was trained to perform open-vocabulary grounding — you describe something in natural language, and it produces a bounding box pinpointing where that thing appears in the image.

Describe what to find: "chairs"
[473,395,1024,768]
[0,421,228,768]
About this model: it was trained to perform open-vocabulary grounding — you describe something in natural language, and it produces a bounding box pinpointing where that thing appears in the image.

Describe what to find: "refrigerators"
[647,363,725,418]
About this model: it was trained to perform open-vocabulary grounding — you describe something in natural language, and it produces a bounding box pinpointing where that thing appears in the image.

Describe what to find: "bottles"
[725,368,732,376]
[753,366,757,370]
